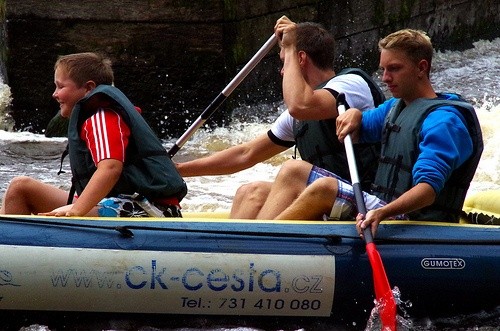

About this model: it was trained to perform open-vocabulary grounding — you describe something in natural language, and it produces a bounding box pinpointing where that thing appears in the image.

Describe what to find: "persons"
[1,51,187,217]
[171,15,390,220]
[255,29,485,242]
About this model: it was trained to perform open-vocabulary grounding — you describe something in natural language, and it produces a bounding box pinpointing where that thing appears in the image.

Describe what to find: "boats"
[0,186,500,331]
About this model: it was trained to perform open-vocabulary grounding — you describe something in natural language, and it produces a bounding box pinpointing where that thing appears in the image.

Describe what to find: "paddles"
[165,31,284,162]
[336,89,397,330]
